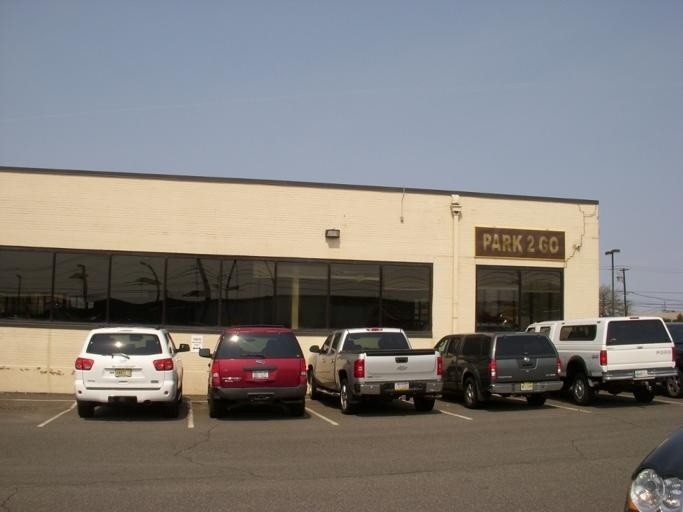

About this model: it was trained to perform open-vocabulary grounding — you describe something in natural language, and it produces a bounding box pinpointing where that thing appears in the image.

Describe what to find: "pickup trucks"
[310,327,443,414]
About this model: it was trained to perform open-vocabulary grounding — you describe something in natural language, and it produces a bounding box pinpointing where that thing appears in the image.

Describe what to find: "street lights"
[605,249,621,314]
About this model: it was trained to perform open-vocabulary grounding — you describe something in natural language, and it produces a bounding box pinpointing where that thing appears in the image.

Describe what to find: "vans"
[437,316,682,408]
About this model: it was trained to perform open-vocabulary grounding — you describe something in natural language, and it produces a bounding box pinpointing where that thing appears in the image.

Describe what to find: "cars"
[73,326,308,415]
[626,430,681,512]
